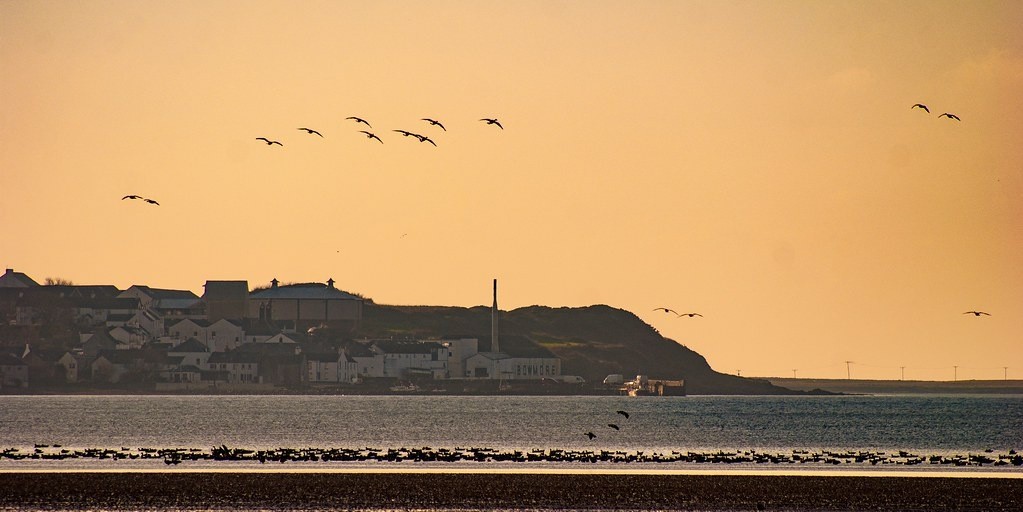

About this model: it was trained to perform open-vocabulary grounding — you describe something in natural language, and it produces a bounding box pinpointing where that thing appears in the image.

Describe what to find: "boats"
[625,376,687,396]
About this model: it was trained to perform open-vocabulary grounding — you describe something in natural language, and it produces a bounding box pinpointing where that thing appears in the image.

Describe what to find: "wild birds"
[346,117,372,129]
[420,118,446,131]
[652,307,703,319]
[256,137,283,147]
[392,129,438,147]
[357,131,383,144]
[297,127,324,138]
[910,103,961,121]
[963,311,992,317]
[584,411,630,440]
[121,194,160,206]
[479,118,504,129]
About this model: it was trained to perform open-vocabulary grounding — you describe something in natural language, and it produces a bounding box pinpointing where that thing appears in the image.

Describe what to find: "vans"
[604,374,623,384]
[563,375,586,382]
